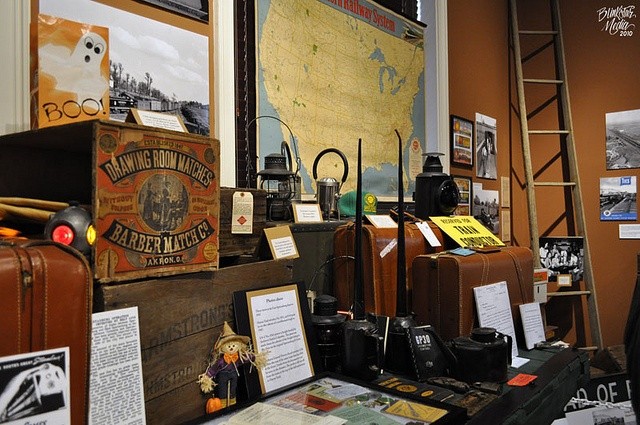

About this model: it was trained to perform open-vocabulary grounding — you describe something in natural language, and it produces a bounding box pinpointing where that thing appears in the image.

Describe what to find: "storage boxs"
[94,256,293,422]
[2,119,221,285]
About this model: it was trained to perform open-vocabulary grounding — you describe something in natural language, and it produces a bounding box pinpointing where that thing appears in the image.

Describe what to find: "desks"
[207,345,591,425]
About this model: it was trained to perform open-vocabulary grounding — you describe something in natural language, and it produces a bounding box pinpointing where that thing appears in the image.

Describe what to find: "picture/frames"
[451,174,473,216]
[450,113,476,170]
[31,0,215,137]
[539,236,586,282]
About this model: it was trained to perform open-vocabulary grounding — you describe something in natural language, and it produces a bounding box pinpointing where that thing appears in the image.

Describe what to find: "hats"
[338,191,378,216]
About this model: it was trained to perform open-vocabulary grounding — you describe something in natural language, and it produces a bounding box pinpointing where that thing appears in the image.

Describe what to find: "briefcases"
[412,247,535,342]
[0,238,93,425]
[333,209,445,320]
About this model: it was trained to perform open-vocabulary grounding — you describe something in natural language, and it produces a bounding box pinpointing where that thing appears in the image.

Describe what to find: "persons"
[550,244,561,262]
[569,253,578,266]
[540,242,549,265]
[544,252,552,269]
[198,320,269,414]
[560,251,568,267]
[552,253,560,269]
[482,131,496,179]
